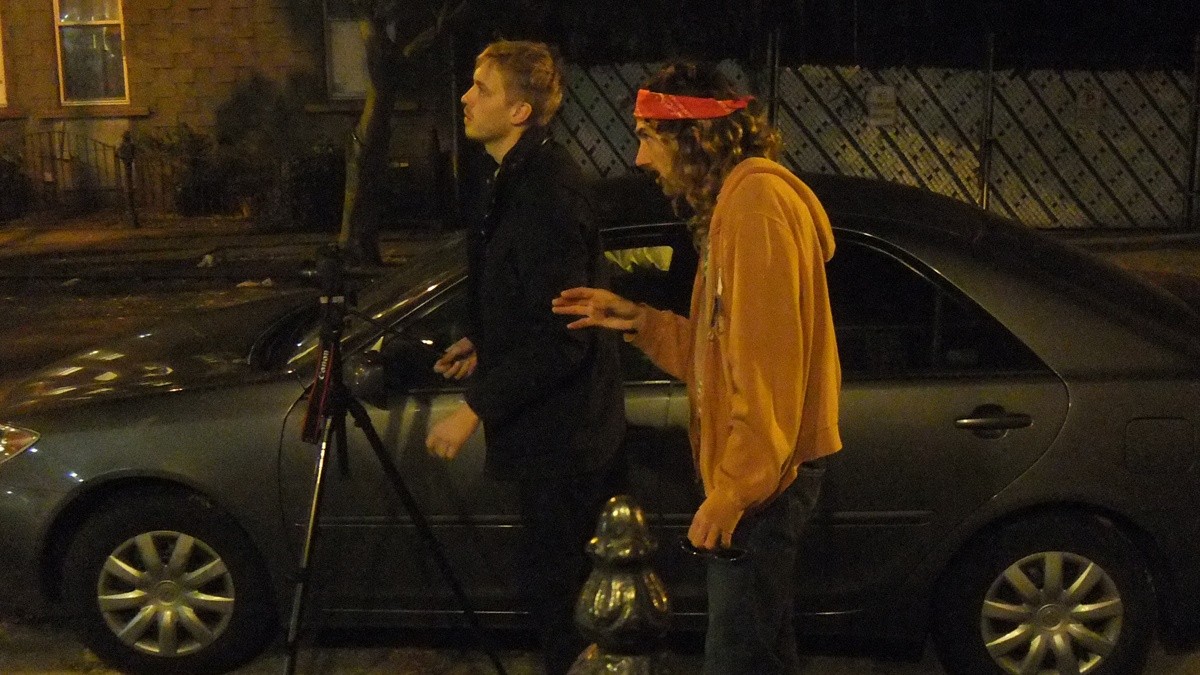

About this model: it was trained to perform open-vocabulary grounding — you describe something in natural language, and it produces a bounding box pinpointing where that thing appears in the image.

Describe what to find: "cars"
[1,176,1200,674]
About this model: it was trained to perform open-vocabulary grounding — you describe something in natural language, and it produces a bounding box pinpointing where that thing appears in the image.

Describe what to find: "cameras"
[298,246,353,293]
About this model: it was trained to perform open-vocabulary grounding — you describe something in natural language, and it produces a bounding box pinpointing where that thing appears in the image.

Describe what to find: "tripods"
[281,292,509,675]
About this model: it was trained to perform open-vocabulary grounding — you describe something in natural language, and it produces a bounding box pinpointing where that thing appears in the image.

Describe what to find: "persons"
[425,36,628,675]
[553,59,843,675]
